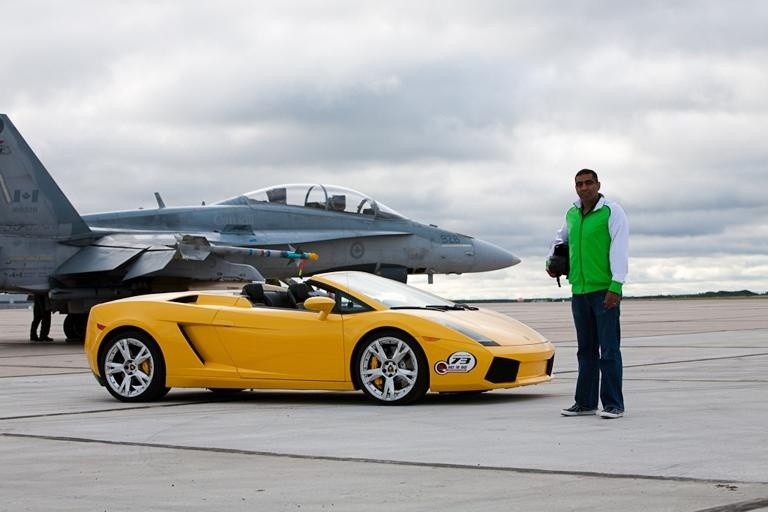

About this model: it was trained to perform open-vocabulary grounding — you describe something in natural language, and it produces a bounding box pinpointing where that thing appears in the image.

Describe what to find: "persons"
[28,290,57,343]
[546,169,628,419]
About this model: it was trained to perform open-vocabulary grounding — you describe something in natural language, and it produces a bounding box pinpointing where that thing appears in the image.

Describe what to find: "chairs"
[241,283,274,309]
[266,188,287,206]
[286,284,319,311]
[332,195,346,212]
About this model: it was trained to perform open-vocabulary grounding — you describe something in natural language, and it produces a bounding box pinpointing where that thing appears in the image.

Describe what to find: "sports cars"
[82,268,556,407]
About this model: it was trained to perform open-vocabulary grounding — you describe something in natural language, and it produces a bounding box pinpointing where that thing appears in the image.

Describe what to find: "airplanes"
[0,113,521,340]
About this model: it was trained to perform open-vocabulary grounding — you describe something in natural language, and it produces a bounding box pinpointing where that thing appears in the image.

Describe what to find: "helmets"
[548,244,569,276]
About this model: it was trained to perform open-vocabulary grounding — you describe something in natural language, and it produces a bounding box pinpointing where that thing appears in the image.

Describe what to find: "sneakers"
[600,410,625,418]
[561,403,598,416]
[30,336,54,342]
